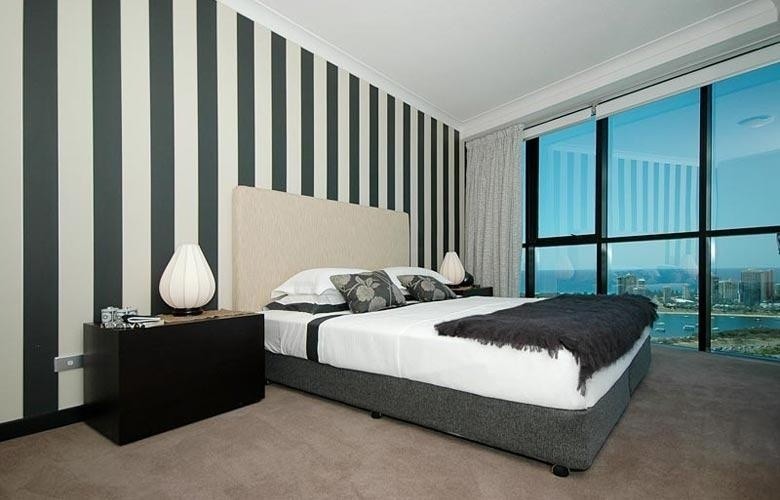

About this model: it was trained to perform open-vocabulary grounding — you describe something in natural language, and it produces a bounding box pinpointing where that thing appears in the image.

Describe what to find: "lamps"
[440,251,466,285]
[158,243,217,317]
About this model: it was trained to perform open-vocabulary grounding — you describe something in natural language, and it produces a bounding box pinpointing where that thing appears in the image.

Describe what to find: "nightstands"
[449,281,493,299]
[81,309,266,447]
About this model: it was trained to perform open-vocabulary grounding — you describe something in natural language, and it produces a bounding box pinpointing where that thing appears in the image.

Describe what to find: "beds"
[231,184,656,476]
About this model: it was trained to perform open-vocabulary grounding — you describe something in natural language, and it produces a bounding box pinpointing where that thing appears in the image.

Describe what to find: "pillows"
[269,266,461,314]
